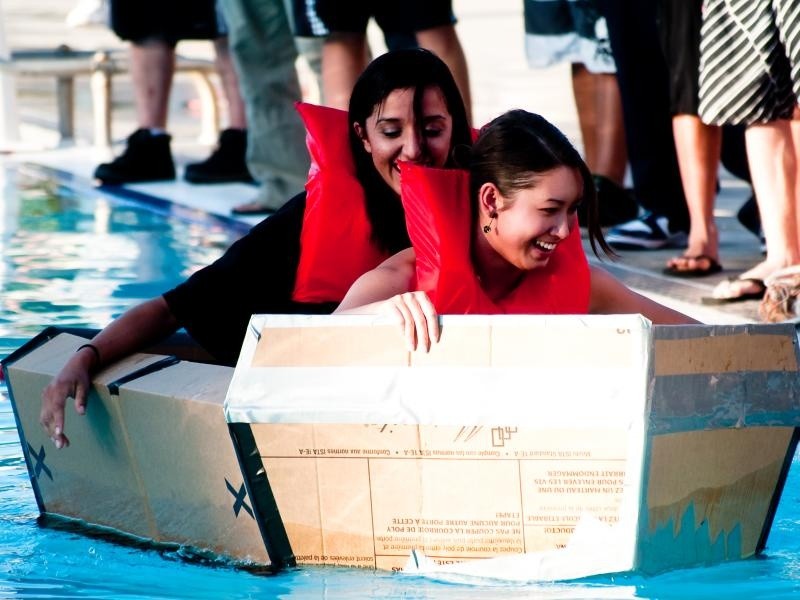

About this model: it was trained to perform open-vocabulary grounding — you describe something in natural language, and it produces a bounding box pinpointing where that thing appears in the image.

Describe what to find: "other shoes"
[575,175,640,226]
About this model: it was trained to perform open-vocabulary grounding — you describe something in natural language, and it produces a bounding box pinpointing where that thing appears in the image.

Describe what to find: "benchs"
[0,49,226,154]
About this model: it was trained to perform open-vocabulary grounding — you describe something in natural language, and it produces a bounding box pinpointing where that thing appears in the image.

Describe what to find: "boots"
[185,127,249,184]
[94,129,175,184]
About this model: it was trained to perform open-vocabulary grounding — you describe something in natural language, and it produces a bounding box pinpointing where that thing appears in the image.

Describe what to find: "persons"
[39,48,482,450]
[0,0,800,325]
[333,107,710,357]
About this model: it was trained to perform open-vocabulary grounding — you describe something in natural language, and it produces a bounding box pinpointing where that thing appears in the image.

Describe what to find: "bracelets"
[75,344,105,373]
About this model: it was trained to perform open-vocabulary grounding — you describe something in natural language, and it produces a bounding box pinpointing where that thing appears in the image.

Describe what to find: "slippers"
[232,201,273,215]
[701,274,766,305]
[663,253,722,277]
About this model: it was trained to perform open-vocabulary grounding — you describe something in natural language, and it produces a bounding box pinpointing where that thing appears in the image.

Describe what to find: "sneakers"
[603,206,690,250]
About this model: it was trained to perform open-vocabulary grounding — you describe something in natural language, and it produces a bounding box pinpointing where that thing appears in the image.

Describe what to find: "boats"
[1,276,800,593]
